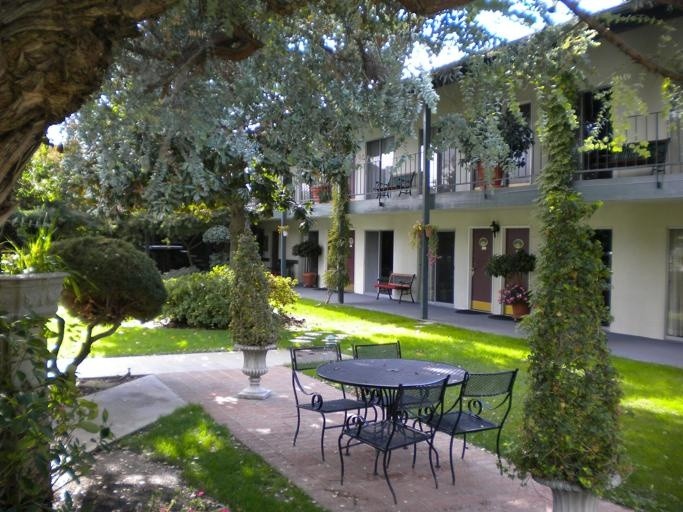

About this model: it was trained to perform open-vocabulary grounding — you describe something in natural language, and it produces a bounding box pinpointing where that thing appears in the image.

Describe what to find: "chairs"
[288,340,519,505]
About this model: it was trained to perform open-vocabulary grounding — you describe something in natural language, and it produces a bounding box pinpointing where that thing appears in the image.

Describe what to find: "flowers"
[498,284,530,304]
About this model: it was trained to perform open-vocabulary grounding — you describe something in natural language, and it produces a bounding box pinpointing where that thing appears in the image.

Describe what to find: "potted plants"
[292,242,321,288]
[497,73,636,511]
[232,216,278,400]
[454,97,534,188]
[0,215,99,323]
[301,142,338,203]
[407,217,439,258]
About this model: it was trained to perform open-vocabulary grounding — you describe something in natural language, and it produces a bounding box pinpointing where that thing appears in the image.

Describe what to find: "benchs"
[572,139,669,189]
[373,171,415,198]
[374,273,415,304]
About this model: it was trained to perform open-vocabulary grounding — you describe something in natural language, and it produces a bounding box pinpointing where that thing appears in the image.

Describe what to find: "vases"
[504,303,529,322]
